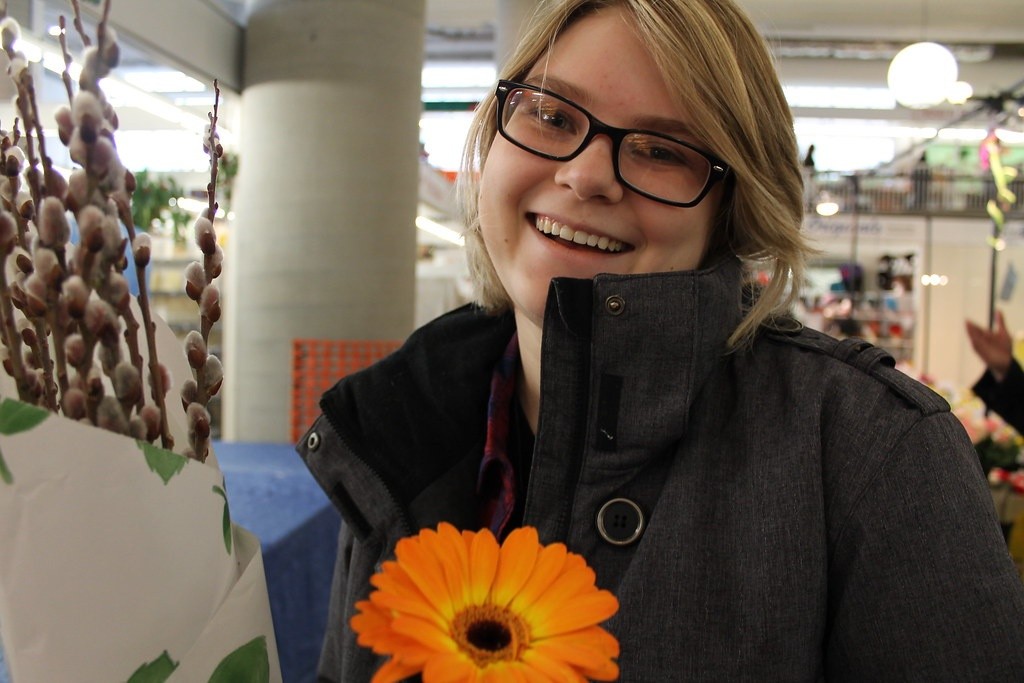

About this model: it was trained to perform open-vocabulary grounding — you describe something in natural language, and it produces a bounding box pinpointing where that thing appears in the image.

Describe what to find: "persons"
[294,0,1024,683]
[0,208,218,475]
[803,146,815,210]
[910,156,932,210]
[965,309,1024,437]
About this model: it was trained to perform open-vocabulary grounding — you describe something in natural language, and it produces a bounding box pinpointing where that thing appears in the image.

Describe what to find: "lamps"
[886,0,960,112]
[815,188,840,218]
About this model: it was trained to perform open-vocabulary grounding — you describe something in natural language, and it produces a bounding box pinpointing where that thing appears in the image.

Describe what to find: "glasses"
[496,79,734,210]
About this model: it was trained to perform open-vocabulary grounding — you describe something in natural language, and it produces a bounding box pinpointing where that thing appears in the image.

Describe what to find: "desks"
[210,436,346,683]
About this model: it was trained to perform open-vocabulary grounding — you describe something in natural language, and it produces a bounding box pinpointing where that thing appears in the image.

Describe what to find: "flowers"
[351,519,621,683]
[0,0,242,467]
